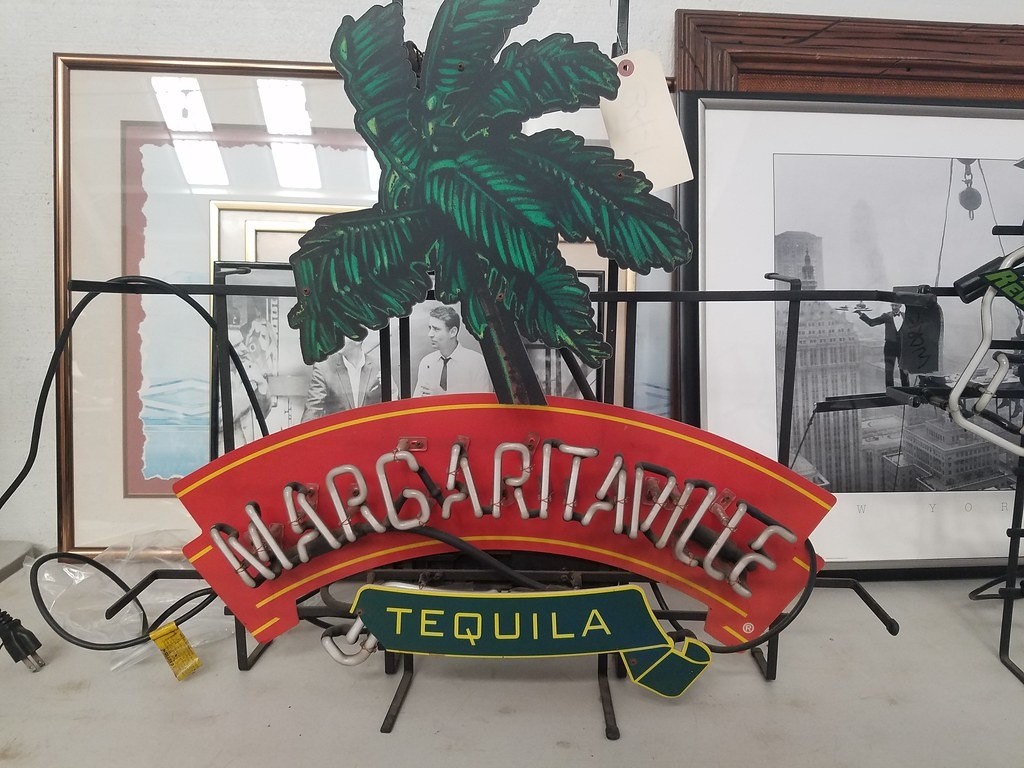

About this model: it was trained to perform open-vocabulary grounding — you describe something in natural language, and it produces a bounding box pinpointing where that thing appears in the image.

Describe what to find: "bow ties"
[893,312,900,317]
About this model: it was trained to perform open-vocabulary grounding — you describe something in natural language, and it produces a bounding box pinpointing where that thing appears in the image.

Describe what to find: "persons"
[300,324,398,423]
[413,306,493,398]
[854,303,910,388]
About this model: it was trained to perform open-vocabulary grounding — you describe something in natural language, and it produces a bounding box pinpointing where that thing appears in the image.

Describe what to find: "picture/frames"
[668,6,1024,421]
[207,200,638,410]
[667,86,1024,584]
[52,51,637,565]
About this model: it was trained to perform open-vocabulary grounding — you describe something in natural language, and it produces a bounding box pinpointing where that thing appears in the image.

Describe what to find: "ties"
[440,356,452,390]
